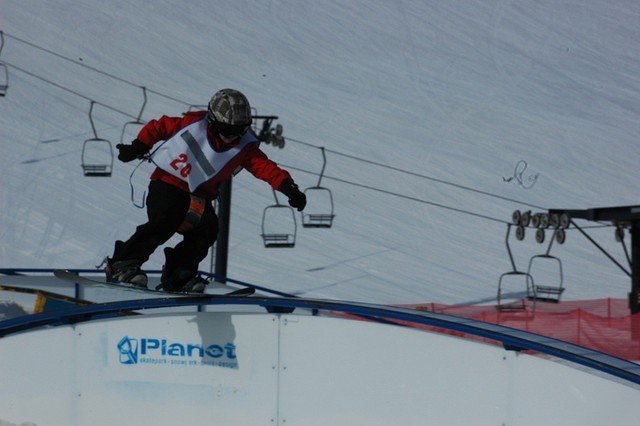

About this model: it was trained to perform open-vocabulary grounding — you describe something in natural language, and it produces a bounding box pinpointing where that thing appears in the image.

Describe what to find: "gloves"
[116,139,151,163]
[280,180,306,211]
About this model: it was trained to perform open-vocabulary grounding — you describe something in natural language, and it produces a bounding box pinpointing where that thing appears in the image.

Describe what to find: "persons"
[102,89,307,295]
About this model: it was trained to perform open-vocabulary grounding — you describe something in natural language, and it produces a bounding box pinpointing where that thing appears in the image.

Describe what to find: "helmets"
[208,88,252,126]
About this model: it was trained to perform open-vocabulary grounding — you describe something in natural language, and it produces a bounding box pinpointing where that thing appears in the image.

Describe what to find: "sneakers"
[161,247,209,293]
[105,240,148,287]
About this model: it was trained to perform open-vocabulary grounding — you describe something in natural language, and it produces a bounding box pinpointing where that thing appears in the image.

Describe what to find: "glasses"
[212,121,249,138]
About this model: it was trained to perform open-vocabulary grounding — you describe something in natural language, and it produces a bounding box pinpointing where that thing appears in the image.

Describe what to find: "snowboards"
[53,269,255,297]
